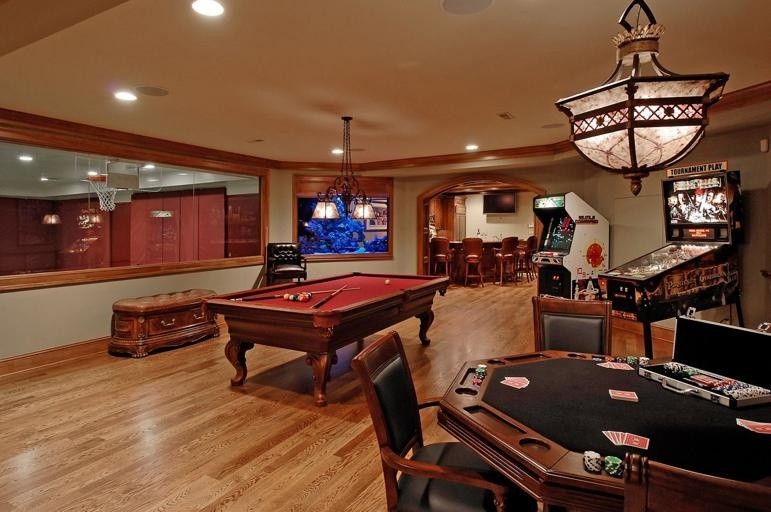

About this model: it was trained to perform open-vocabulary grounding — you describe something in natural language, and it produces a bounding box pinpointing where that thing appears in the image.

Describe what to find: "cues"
[311,284,349,309]
[241,288,361,301]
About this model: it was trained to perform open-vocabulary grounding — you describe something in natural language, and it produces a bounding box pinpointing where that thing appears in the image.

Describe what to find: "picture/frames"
[364,201,388,232]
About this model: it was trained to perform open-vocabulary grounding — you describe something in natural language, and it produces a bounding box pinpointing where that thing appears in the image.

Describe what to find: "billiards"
[384,279,391,284]
[283,290,311,302]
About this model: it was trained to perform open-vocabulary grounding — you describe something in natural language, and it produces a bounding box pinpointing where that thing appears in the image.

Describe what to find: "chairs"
[623,450,771,512]
[490,236,519,286]
[531,295,613,356]
[429,237,455,277]
[511,236,538,283]
[351,330,541,512]
[266,241,307,284]
[462,238,485,288]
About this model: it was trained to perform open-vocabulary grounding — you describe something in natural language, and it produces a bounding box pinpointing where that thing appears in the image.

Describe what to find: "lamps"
[43,214,61,226]
[311,116,377,220]
[555,1,730,197]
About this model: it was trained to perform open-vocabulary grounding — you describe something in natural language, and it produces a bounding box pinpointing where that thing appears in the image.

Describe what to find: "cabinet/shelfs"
[427,195,466,241]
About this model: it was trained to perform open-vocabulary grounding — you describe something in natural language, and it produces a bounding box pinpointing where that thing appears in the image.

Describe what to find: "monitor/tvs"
[542,215,575,253]
[482,193,517,215]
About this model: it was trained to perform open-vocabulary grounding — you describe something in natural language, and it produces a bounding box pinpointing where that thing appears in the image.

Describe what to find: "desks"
[108,288,220,358]
[436,348,771,512]
[448,241,502,284]
[205,270,450,407]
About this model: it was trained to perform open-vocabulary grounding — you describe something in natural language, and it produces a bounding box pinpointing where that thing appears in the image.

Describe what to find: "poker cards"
[499,374,529,390]
[602,430,650,449]
[598,361,633,370]
[609,390,640,402]
[737,418,771,434]
